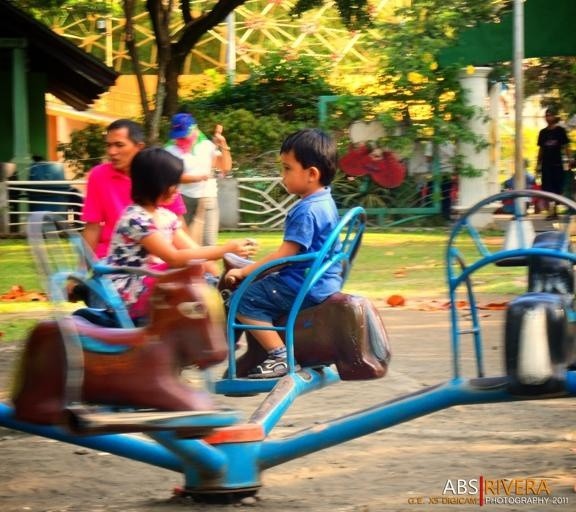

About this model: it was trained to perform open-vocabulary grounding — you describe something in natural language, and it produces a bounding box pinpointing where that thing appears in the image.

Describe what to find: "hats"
[168,113,194,138]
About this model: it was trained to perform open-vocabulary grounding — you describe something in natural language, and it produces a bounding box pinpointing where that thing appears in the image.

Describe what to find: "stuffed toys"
[9,230,394,425]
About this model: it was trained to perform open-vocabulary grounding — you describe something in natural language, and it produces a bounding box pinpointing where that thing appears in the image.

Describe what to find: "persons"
[534,107,574,221]
[158,113,234,247]
[101,147,259,322]
[68,118,223,326]
[219,128,346,379]
[494,158,536,215]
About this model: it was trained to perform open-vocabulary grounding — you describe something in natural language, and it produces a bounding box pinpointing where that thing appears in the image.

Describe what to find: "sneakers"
[545,214,559,220]
[495,207,503,213]
[249,355,300,378]
